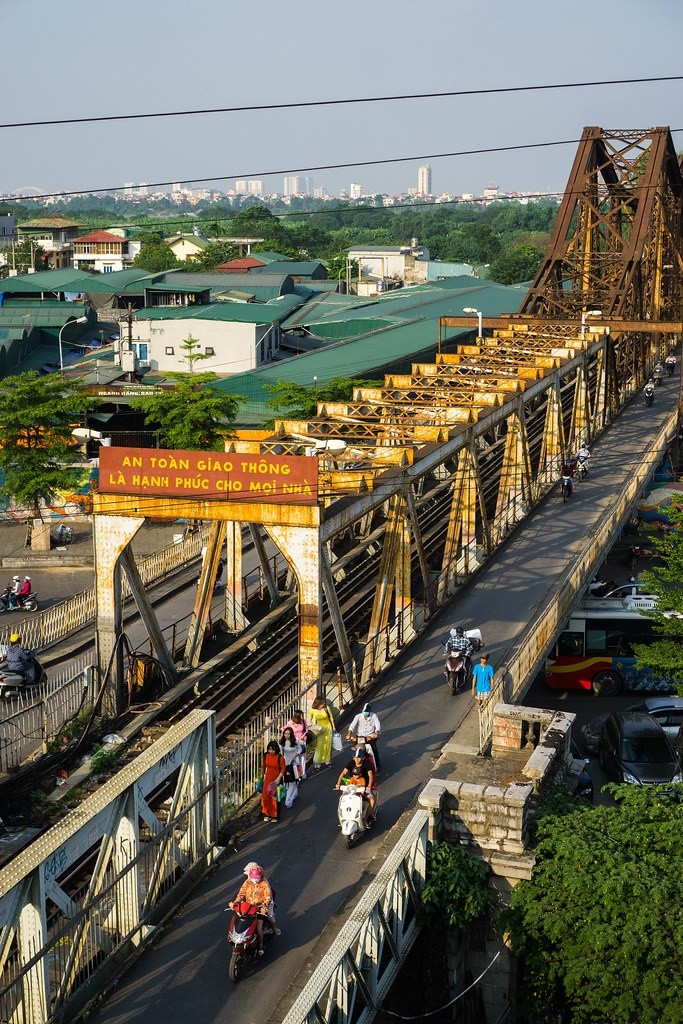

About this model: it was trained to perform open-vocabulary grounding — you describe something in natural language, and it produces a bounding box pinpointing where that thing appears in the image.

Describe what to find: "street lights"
[463,307,482,338]
[581,310,604,341]
[339,266,353,294]
[58,316,88,371]
[463,263,490,279]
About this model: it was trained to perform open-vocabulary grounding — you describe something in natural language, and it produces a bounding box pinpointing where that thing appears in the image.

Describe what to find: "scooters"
[0,649,47,704]
[196,559,227,591]
[347,734,377,777]
[331,784,380,850]
[651,366,663,388]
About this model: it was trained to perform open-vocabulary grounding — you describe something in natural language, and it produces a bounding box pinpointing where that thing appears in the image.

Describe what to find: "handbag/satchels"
[284,764,295,782]
[276,785,287,804]
[301,745,306,754]
[332,733,342,751]
[257,779,263,793]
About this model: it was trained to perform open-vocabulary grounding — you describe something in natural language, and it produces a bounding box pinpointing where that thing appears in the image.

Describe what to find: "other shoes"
[275,928,281,936]
[370,814,377,822]
[363,820,371,829]
[271,818,278,822]
[259,950,264,956]
[264,817,271,821]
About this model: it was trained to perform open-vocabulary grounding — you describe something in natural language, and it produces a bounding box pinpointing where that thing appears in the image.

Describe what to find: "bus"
[545,595,683,699]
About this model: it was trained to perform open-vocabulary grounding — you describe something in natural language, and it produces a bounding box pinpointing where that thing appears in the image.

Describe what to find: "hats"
[24,576,32,582]
[481,653,490,659]
[351,743,366,752]
[351,767,362,775]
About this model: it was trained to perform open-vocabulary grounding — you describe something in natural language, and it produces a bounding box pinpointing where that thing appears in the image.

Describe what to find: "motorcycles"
[225,889,277,984]
[440,642,472,696]
[0,582,39,613]
[560,471,577,504]
[665,360,677,378]
[643,386,654,408]
[575,454,592,484]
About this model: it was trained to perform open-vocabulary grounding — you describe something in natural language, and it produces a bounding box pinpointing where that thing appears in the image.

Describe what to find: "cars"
[587,574,658,599]
[583,696,683,751]
[567,737,594,804]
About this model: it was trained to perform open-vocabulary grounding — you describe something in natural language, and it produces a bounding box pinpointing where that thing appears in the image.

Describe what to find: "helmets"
[11,634,22,643]
[581,444,586,448]
[13,576,20,581]
[362,703,372,712]
[250,866,264,879]
[567,459,572,463]
[456,627,463,634]
[354,748,365,759]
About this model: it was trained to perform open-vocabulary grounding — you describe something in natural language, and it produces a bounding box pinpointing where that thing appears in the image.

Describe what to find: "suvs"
[600,710,683,805]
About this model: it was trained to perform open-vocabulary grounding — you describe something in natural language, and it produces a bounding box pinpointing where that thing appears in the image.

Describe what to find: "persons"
[10,576,31,607]
[445,627,474,679]
[260,741,286,822]
[336,742,378,829]
[308,696,336,771]
[6,634,34,671]
[575,444,591,468]
[282,710,309,779]
[346,703,382,776]
[278,727,303,808]
[643,379,655,401]
[560,459,576,493]
[652,360,663,372]
[665,352,676,363]
[472,653,494,706]
[229,862,281,955]
